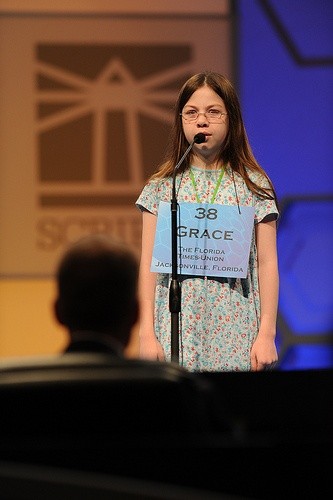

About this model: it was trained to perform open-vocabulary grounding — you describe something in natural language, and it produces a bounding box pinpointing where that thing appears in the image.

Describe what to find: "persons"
[53,237,141,365]
[132,70,285,373]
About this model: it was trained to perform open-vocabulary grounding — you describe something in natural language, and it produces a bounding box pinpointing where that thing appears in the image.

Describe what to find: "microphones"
[173,132,206,171]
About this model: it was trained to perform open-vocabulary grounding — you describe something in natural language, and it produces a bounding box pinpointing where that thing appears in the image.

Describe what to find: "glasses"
[179,110,228,121]
